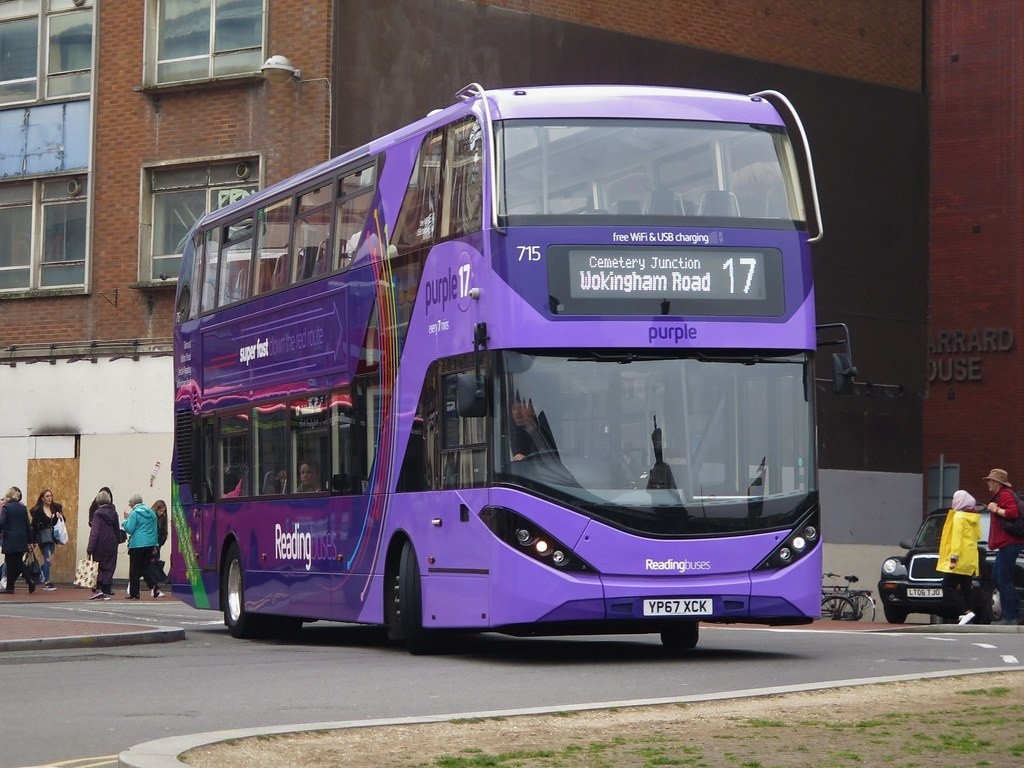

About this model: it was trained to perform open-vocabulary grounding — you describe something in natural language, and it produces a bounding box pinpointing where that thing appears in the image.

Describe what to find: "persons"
[298,459,322,492]
[222,477,243,498]
[122,495,160,599]
[86,487,119,601]
[28,490,66,591]
[983,468,1024,626]
[127,501,168,598]
[263,462,288,495]
[0,487,36,594]
[936,490,982,625]
[505,387,555,462]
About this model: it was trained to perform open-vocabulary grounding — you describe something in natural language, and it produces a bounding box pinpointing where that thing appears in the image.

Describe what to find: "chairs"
[204,237,346,309]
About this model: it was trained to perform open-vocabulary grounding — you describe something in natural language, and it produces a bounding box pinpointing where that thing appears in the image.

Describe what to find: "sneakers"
[126,580,131,594]
[39,565,46,582]
[151,589,165,597]
[957,612,975,626]
[43,582,57,591]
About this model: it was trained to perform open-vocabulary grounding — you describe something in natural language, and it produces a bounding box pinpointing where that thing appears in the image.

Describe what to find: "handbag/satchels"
[994,488,1024,539]
[40,512,53,544]
[73,553,99,588]
[21,543,45,579]
[117,530,127,544]
[54,515,69,544]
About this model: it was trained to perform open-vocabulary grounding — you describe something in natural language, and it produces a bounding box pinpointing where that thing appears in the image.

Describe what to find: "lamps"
[260,55,302,84]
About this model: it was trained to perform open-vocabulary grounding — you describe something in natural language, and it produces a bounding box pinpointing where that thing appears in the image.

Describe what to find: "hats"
[983,469,1012,487]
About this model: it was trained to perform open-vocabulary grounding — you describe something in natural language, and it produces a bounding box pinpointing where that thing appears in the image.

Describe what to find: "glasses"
[159,508,165,512]
[128,504,134,506]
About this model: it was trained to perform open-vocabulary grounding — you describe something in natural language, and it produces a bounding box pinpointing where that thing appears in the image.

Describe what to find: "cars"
[878,506,1024,624]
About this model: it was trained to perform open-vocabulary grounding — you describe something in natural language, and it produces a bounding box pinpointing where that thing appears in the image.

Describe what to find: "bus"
[171,82,860,656]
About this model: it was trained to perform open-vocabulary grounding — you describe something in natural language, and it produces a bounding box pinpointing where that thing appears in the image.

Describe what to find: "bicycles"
[819,571,877,623]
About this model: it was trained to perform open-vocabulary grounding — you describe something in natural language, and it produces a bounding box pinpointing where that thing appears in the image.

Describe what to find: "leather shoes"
[991,617,1018,625]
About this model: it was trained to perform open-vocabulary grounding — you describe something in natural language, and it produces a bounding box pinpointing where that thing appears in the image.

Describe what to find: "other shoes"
[90,589,103,599]
[95,594,111,601]
[1,577,7,589]
[153,584,162,598]
[28,580,36,594]
[110,591,114,595]
[0,589,14,594]
[124,592,140,599]
